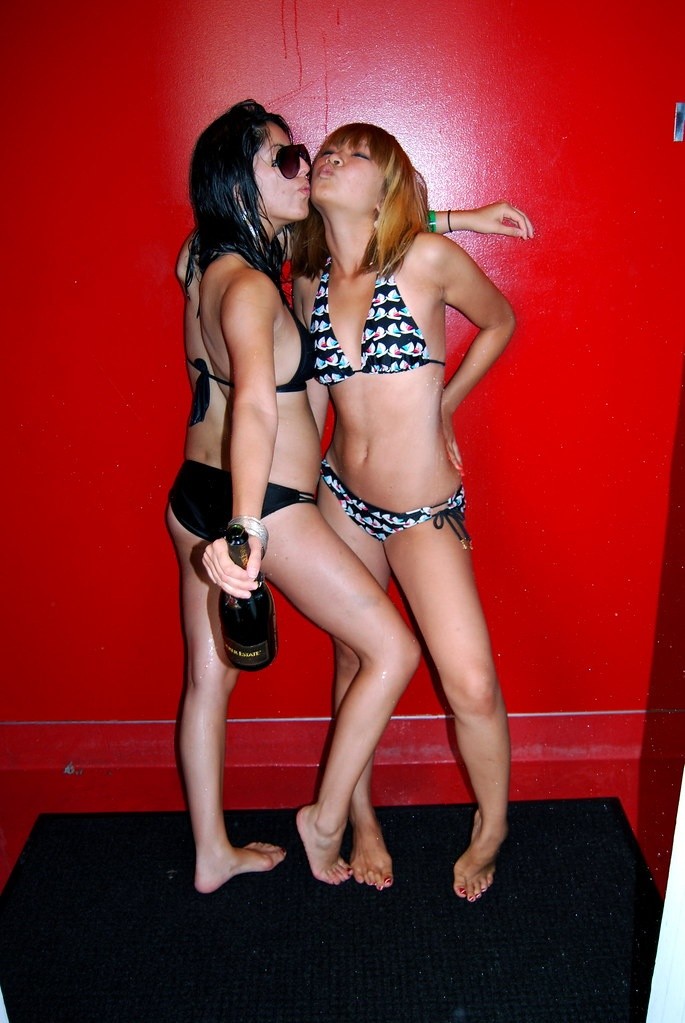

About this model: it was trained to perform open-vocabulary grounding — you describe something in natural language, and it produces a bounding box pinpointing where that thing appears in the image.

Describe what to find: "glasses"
[272,143,313,179]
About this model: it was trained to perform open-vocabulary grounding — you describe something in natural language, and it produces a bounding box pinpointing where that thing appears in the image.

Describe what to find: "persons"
[291,124,516,902]
[163,98,536,893]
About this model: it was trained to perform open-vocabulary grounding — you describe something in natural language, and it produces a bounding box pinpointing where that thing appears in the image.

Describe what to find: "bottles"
[218,524,277,671]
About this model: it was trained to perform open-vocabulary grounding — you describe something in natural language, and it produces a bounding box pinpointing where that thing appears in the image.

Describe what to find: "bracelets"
[426,210,436,233]
[229,516,268,560]
[448,210,453,233]
